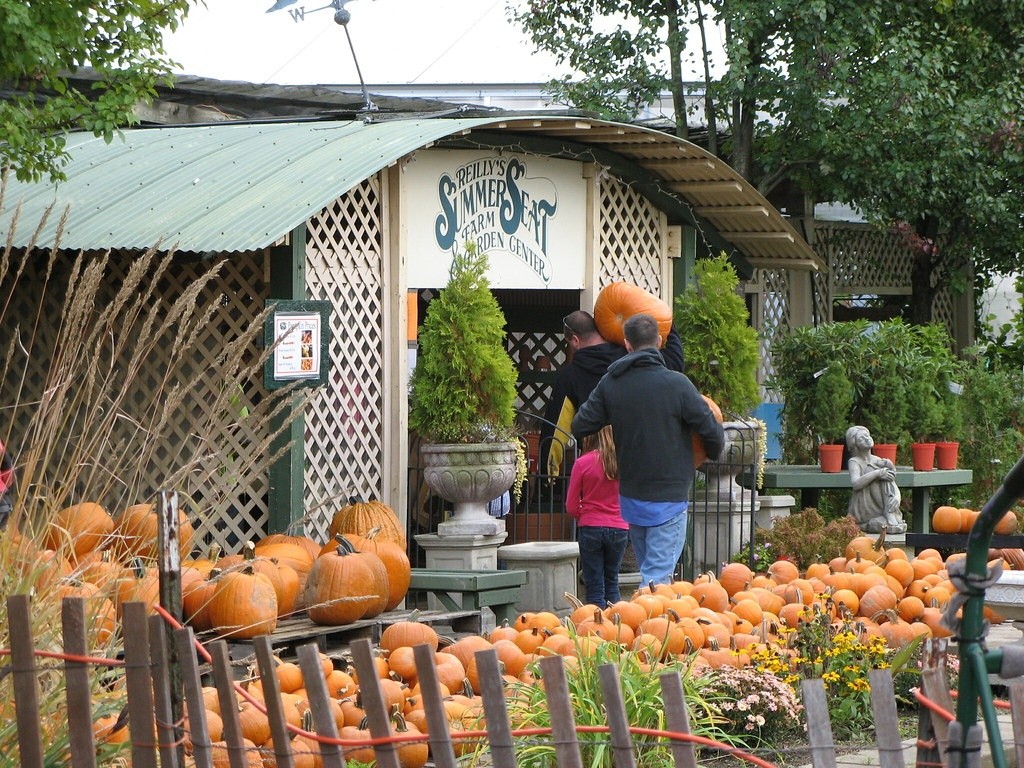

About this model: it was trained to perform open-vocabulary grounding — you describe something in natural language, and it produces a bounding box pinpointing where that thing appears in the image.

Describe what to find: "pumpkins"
[932,507,1018,534]
[0,526,1024,768]
[594,282,673,348]
[692,395,722,468]
[0,496,411,651]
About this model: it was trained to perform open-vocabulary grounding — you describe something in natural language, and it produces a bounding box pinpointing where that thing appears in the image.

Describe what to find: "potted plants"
[666,251,768,502]
[409,242,529,535]
[772,321,964,470]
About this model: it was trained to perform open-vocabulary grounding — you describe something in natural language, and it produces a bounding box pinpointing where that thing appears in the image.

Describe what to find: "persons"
[485,489,510,570]
[566,424,630,612]
[538,311,684,585]
[846,426,903,526]
[571,314,725,588]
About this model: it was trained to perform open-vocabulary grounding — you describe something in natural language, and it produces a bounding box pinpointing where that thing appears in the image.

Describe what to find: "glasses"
[563,316,578,335]
